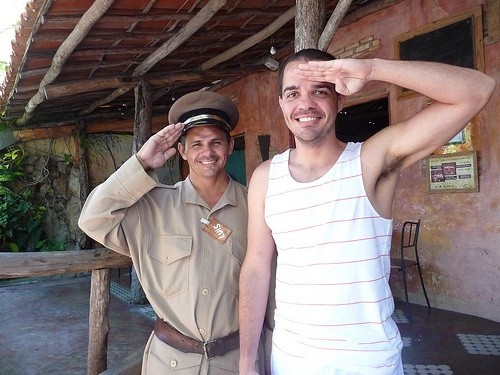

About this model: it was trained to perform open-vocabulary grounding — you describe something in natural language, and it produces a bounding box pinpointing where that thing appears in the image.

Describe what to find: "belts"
[154,315,265,360]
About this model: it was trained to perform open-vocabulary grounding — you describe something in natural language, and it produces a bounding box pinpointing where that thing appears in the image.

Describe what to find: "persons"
[78,91,277,375]
[238,49,497,375]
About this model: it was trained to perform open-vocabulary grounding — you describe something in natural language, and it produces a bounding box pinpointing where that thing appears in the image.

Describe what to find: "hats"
[168,92,239,140]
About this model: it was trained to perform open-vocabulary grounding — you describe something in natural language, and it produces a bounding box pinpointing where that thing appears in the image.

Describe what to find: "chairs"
[391,219,432,324]
